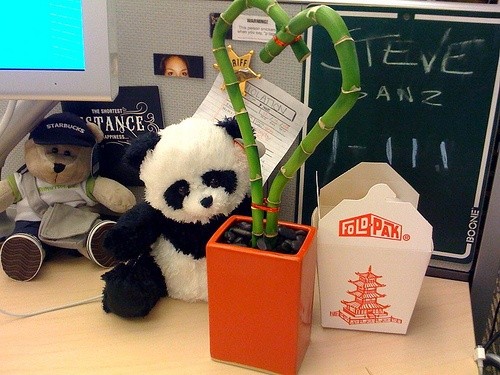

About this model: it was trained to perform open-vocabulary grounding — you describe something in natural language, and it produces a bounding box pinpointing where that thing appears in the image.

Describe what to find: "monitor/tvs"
[0,1,119,251]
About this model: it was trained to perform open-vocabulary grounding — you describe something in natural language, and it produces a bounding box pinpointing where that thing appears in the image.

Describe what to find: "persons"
[161,55,190,77]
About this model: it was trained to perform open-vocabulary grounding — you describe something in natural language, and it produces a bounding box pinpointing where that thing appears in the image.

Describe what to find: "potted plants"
[205,0,362,375]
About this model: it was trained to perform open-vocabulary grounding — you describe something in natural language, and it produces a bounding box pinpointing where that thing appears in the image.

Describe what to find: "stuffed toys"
[0,112,138,283]
[101,116,266,319]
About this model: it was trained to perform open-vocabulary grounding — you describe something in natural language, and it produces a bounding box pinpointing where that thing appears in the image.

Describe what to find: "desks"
[0,183,479,375]
